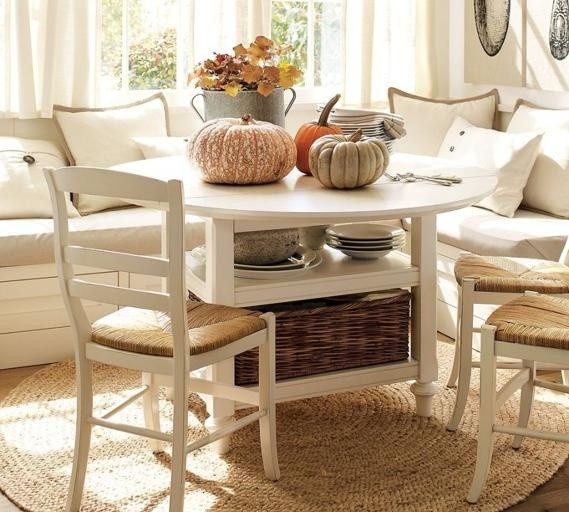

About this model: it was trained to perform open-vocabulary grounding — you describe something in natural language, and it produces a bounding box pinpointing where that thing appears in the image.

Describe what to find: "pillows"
[57,94,170,217]
[436,116,546,216]
[0,137,79,221]
[504,100,568,221]
[390,88,498,157]
[133,136,191,162]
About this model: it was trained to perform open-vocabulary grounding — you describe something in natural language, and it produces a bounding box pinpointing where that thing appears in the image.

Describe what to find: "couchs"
[406,87,568,355]
[2,107,205,367]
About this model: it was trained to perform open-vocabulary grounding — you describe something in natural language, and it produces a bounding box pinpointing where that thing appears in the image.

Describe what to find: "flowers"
[186,36,304,97]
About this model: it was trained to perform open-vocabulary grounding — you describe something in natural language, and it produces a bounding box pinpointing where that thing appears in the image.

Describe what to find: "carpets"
[1,337,568,512]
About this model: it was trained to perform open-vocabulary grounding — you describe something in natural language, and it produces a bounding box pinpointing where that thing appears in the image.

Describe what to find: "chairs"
[466,295,569,505]
[445,230,568,434]
[39,166,280,511]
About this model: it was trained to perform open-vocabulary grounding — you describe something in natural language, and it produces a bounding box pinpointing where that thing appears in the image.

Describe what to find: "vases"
[191,86,297,130]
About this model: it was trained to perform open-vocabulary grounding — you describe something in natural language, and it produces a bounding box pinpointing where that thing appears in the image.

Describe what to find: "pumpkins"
[293,94,341,178]
[185,113,298,184]
[303,128,393,190]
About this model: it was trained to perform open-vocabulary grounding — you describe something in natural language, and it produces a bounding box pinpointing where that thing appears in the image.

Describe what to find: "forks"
[398,170,452,187]
[384,170,463,191]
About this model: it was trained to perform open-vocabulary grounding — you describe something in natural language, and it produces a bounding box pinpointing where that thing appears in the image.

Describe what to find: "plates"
[233,247,317,270]
[330,120,395,158]
[324,222,407,260]
[232,254,324,281]
